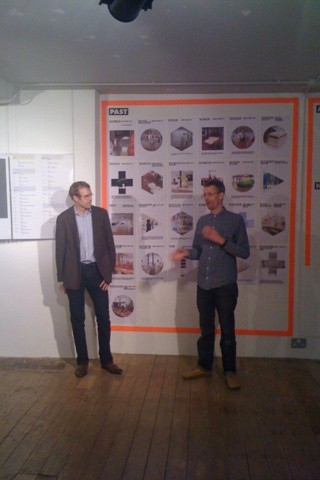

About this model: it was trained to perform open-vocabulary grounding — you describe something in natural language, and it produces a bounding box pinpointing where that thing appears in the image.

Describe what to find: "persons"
[169,176,251,392]
[53,180,125,380]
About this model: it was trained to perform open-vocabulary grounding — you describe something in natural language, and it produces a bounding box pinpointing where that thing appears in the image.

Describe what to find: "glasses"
[201,191,220,199]
[77,194,94,199]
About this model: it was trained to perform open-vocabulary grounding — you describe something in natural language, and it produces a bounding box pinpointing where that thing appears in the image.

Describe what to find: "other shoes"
[101,363,123,373]
[225,371,240,389]
[182,368,211,379]
[76,364,88,378]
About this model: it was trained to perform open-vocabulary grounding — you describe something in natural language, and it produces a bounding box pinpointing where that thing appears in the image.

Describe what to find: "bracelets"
[219,238,229,249]
